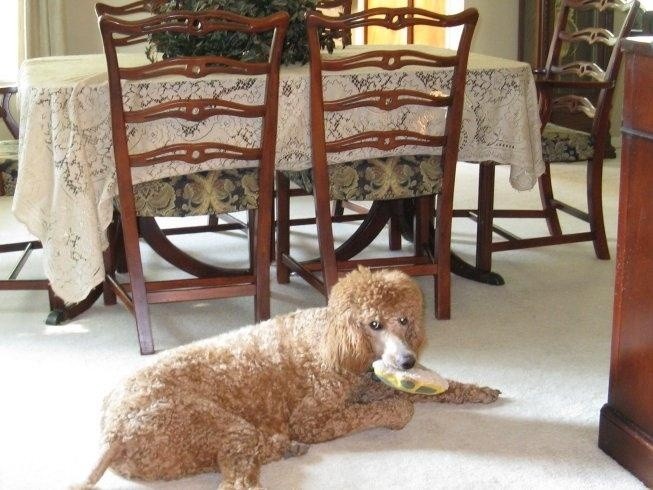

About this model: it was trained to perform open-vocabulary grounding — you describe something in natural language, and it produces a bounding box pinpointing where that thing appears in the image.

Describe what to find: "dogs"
[72,263,503,490]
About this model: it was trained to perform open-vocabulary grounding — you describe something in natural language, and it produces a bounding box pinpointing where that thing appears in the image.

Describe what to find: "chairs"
[96,0,479,355]
[457,1,639,276]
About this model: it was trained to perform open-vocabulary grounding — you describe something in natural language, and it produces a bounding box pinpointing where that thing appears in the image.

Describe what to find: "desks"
[22,45,529,325]
